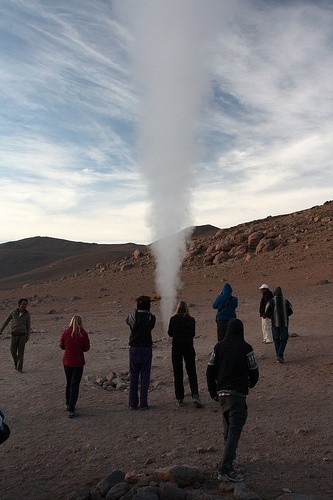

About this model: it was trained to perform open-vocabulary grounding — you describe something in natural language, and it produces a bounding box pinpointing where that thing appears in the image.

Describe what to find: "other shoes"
[176,399,185,407]
[129,404,139,410]
[66,411,80,418]
[218,468,245,482]
[277,357,284,363]
[140,404,148,409]
[193,395,203,408]
[16,369,23,374]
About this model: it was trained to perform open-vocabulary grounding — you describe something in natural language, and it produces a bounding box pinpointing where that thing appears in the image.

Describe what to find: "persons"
[259,284,273,344]
[205,318,259,482]
[167,300,203,409]
[212,282,239,342]
[125,295,156,410]
[0,298,31,374]
[59,314,90,418]
[265,286,294,363]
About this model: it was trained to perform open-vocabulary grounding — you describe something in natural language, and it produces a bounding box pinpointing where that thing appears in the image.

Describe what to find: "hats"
[259,283,269,290]
